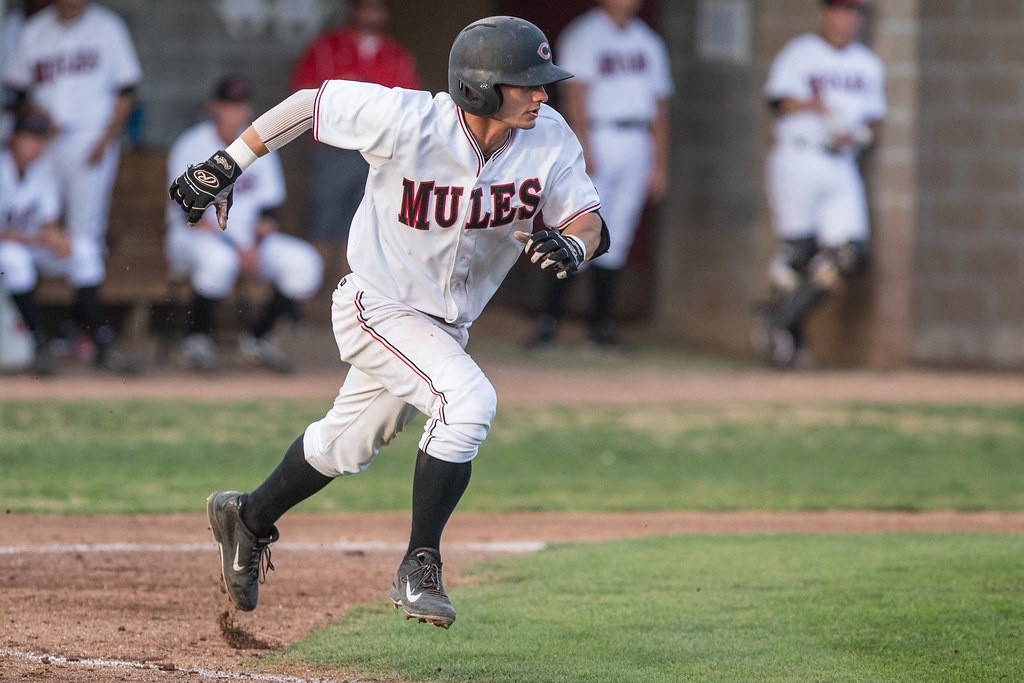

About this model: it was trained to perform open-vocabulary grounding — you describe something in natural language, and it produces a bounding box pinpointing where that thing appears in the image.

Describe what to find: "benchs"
[26,142,308,377]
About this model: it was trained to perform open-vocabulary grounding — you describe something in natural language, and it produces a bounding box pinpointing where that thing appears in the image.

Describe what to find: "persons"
[529,0,675,351]
[754,1,891,373]
[165,75,322,371]
[169,17,610,629]
[0,0,144,375]
[288,0,421,316]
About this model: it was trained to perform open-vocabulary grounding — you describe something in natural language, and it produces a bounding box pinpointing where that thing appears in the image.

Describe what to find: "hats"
[211,74,255,101]
[19,103,75,136]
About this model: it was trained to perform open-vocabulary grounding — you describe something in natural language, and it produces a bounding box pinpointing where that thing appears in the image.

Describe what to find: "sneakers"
[206,490,279,613]
[390,547,457,630]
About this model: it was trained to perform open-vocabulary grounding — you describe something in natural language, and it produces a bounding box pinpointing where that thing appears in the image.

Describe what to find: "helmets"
[449,16,576,117]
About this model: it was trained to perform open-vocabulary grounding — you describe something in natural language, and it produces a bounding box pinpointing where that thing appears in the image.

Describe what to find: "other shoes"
[33,336,58,371]
[762,321,805,369]
[748,301,775,352]
[586,322,623,346]
[86,333,114,368]
[523,318,559,346]
[176,336,220,369]
[236,333,291,369]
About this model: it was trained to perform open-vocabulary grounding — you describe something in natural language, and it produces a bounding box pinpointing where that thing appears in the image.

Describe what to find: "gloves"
[513,228,585,280]
[167,150,242,230]
